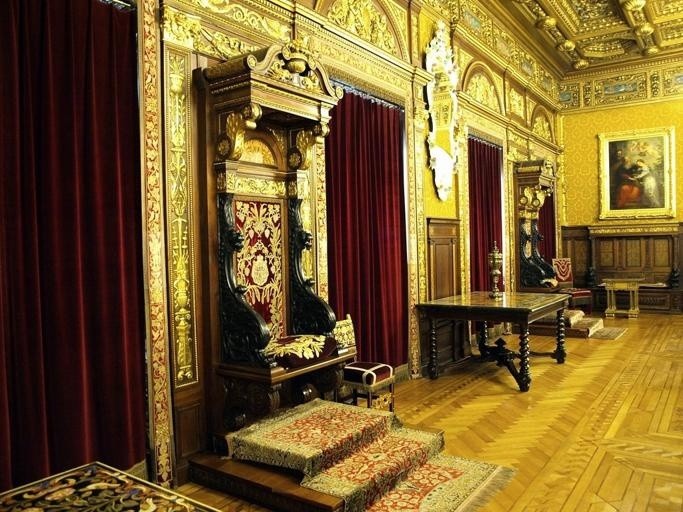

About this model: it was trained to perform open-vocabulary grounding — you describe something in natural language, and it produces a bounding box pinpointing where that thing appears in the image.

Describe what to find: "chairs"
[552,258,593,317]
[190,39,357,424]
[328,315,395,415]
[513,158,562,292]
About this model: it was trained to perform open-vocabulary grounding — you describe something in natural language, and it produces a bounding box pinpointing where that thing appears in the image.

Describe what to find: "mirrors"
[424,22,458,198]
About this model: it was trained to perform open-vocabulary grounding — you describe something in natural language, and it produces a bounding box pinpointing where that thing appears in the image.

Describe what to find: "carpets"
[537,308,628,341]
[226,398,513,512]
[0,461,224,512]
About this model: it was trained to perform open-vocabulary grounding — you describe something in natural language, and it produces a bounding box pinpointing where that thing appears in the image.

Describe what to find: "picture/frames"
[597,125,676,219]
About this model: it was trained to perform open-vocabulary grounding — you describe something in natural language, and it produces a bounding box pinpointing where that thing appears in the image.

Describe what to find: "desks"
[415,291,572,391]
[603,278,643,317]
